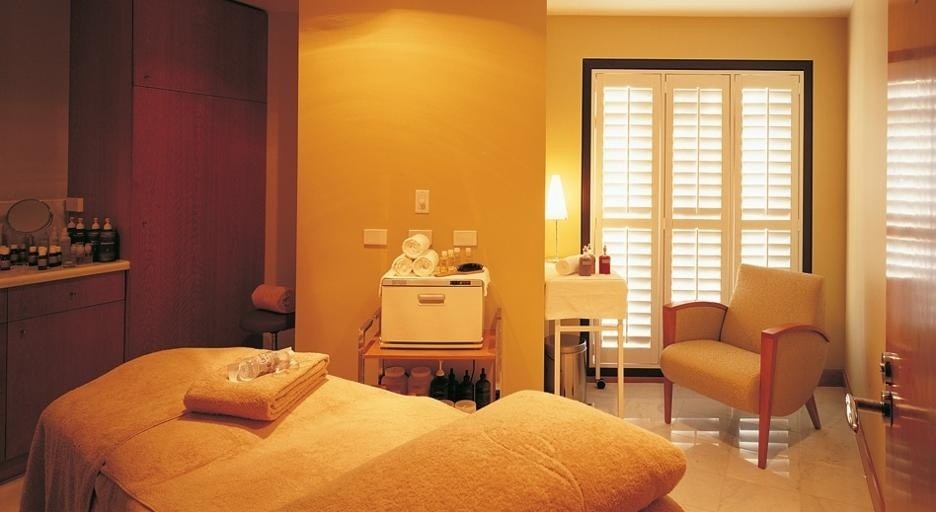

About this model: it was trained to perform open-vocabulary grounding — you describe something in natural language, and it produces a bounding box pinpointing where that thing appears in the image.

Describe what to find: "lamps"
[547,175,568,264]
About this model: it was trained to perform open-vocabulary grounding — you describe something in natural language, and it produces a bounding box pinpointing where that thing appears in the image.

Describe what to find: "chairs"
[662,264,828,468]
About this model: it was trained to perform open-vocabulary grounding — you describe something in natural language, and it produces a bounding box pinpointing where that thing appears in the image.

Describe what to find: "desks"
[545,261,627,421]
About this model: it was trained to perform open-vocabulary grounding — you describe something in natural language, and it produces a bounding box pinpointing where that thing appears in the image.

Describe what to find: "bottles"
[381,360,492,413]
[0,218,119,270]
[578,244,611,276]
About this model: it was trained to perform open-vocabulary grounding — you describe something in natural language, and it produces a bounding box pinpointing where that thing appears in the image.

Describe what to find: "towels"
[402,234,431,258]
[412,250,440,276]
[391,255,412,275]
[183,352,329,423]
[252,284,295,313]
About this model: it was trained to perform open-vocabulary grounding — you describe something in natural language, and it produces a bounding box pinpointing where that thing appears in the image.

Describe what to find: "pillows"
[281,388,688,511]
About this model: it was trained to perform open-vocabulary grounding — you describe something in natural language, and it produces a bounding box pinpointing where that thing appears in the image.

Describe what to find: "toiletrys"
[439,247,473,273]
[0,216,118,271]
[579,244,610,276]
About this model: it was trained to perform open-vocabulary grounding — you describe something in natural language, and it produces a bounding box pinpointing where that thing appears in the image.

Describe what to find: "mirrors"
[7,198,52,234]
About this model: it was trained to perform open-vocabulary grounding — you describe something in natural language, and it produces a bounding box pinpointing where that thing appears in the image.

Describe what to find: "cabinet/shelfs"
[1,261,126,483]
[357,305,501,412]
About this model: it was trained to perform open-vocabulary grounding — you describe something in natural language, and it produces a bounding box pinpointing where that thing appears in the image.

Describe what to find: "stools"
[239,310,295,353]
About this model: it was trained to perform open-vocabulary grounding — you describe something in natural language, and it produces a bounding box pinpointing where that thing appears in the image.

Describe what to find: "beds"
[19,347,686,511]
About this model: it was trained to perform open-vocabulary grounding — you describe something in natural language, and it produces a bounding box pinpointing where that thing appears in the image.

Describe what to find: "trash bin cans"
[544,334,587,402]
